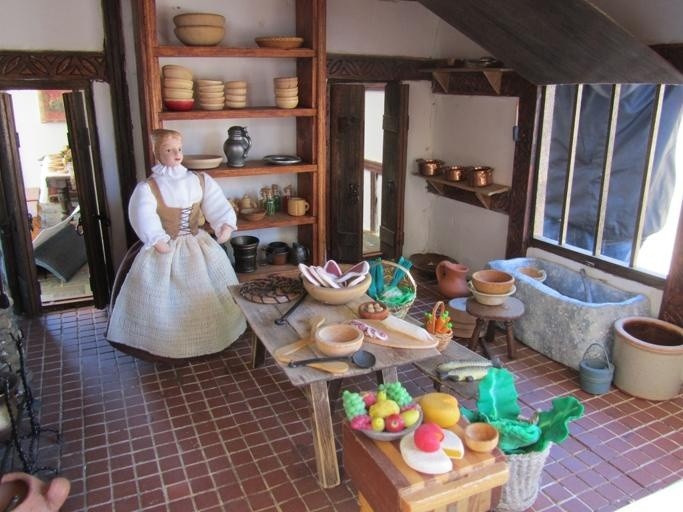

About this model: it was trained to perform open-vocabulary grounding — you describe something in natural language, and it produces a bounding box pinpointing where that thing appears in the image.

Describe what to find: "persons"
[102,128,247,365]
